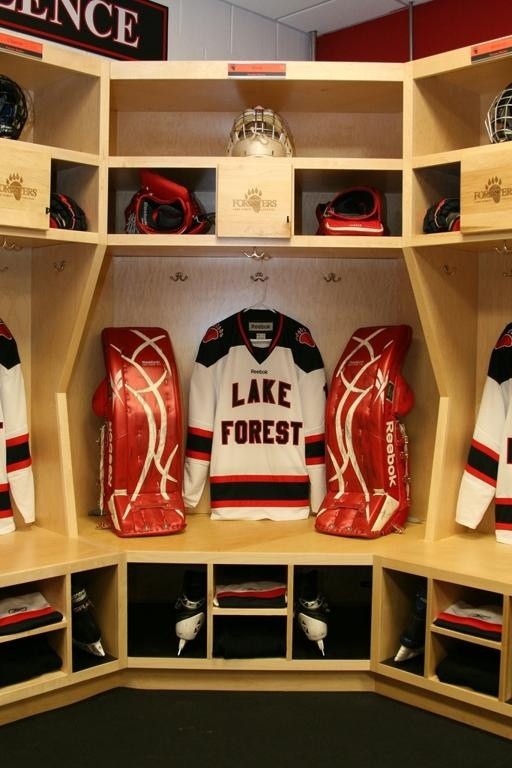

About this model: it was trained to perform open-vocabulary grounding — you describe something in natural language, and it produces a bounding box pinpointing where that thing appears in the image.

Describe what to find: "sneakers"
[297,577,331,639]
[177,577,204,642]
[402,596,425,646]
[71,590,103,648]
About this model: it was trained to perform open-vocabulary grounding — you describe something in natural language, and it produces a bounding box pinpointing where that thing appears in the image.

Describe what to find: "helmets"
[485,82,512,142]
[226,106,293,156]
[0,76,29,139]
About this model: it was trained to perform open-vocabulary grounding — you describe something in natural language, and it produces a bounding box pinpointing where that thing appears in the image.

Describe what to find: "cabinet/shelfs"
[1,32,512,741]
